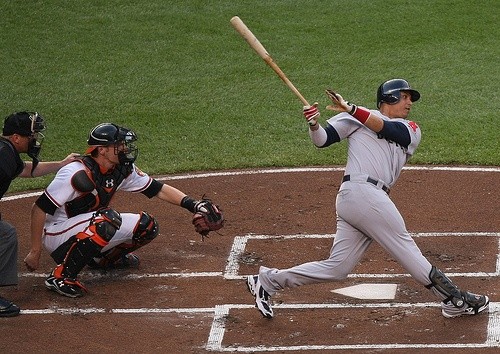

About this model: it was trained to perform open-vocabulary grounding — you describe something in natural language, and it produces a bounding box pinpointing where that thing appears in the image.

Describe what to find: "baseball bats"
[229,14,319,117]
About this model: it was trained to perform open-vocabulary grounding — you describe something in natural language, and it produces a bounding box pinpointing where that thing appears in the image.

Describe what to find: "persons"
[246,78,490,319]
[0,111,80,317]
[23,123,225,298]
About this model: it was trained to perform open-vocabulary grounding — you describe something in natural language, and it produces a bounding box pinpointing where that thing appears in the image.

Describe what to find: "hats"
[2,112,47,135]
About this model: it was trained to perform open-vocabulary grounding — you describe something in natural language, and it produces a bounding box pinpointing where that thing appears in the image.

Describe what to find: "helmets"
[376,78,421,110]
[84,123,138,166]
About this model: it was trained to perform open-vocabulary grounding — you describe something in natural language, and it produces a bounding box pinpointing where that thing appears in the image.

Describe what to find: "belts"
[341,174,391,195]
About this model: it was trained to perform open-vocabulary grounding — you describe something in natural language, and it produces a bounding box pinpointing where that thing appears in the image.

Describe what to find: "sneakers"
[88,252,139,268]
[0,298,20,318]
[246,273,274,318]
[439,291,490,318]
[45,275,83,297]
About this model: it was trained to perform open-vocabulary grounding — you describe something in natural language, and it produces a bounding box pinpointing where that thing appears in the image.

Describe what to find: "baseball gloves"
[191,202,227,237]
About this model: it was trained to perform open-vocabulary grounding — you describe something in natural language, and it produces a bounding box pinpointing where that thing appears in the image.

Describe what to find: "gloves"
[302,102,321,127]
[324,89,357,115]
